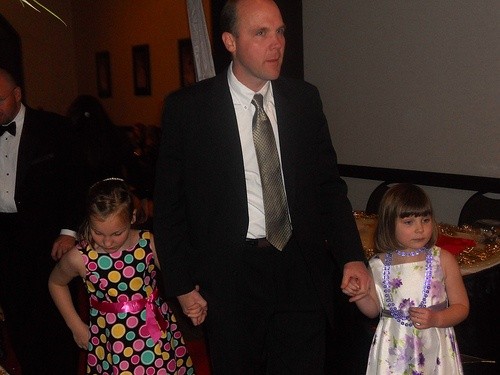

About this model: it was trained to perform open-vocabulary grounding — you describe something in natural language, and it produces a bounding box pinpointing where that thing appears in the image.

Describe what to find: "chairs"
[453,181,500,375]
[365,179,407,215]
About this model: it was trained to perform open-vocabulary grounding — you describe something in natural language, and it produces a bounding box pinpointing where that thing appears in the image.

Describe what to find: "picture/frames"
[131,42,153,97]
[177,36,198,89]
[95,49,114,99]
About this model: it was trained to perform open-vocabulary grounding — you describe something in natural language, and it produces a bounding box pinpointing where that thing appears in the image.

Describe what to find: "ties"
[251,94,293,251]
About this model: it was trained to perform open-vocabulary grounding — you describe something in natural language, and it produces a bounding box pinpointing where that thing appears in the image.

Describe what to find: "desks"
[352,209,500,375]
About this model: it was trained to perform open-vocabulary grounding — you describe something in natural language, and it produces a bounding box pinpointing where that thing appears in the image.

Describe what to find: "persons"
[150,0,371,375]
[66,95,155,229]
[342,185,470,375]
[48,175,206,375]
[0,64,92,375]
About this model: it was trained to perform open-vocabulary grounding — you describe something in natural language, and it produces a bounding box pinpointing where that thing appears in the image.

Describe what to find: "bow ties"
[0,121,16,137]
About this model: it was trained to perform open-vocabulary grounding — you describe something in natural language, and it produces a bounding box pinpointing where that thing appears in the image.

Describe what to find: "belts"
[245,238,273,250]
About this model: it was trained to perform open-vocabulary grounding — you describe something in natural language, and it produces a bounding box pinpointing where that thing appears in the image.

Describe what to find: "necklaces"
[380,246,433,327]
